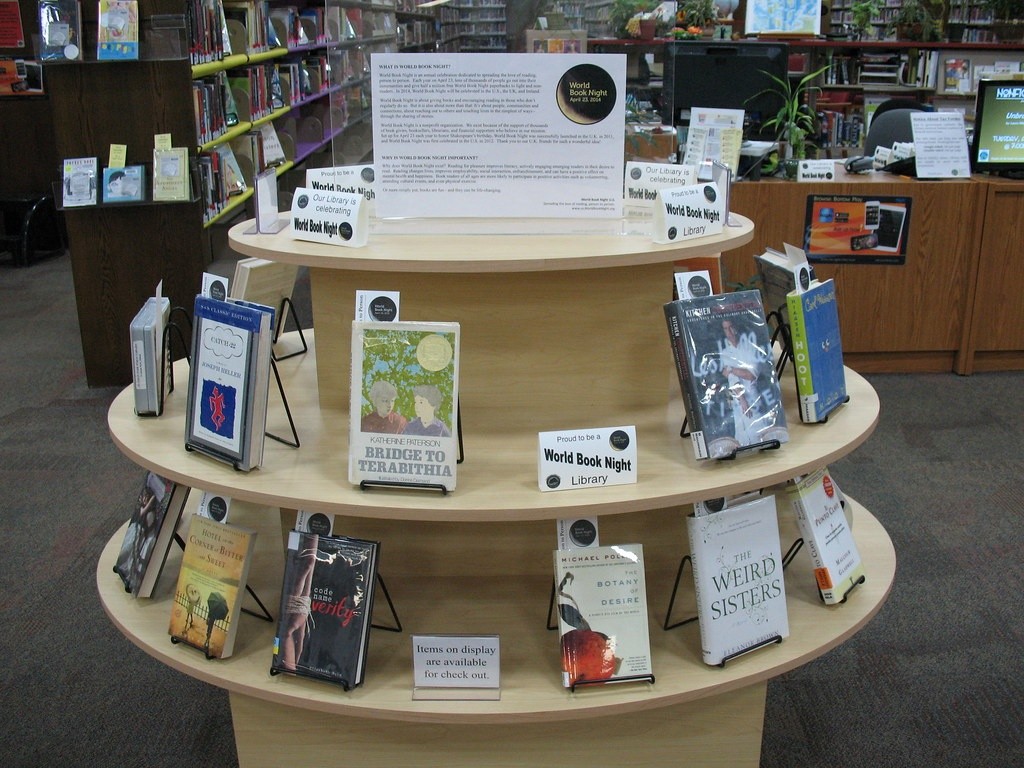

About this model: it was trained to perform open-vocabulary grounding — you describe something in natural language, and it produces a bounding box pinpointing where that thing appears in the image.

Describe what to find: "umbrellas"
[185,582,202,605]
[207,591,228,620]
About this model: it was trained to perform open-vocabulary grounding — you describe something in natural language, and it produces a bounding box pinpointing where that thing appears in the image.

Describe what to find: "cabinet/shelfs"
[36,2,432,390]
[437,0,1024,160]
[94,205,896,766]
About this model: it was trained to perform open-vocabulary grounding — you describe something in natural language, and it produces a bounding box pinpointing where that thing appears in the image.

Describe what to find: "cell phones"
[865,201,880,230]
[851,233,880,250]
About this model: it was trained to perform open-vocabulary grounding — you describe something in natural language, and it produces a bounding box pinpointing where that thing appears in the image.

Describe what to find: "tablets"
[868,205,907,253]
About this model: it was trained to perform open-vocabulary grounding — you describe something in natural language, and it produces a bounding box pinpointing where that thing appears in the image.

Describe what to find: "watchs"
[728,366,733,373]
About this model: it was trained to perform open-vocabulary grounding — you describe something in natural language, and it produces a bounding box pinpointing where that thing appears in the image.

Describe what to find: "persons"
[204,614,215,647]
[720,316,768,447]
[185,601,194,631]
[281,534,319,676]
[116,487,158,598]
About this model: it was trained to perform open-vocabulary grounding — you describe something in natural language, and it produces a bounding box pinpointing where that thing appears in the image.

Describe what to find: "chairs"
[865,97,928,157]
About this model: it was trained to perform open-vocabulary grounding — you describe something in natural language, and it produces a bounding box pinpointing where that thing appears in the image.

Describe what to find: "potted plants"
[887,0,940,42]
[745,61,839,176]
[981,0,1024,44]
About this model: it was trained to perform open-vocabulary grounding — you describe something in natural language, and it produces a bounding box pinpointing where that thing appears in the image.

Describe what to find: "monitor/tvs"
[663,40,790,143]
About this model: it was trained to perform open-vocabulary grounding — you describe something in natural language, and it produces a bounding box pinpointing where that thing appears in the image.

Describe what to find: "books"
[272,529,381,689]
[522,0,1024,182]
[752,246,847,423]
[348,320,460,491]
[553,543,652,687]
[184,257,297,472]
[97,0,139,60]
[190,0,392,224]
[685,491,789,666]
[169,514,258,659]
[396,0,507,53]
[63,147,189,207]
[785,466,865,605]
[663,290,790,461]
[116,470,192,598]
